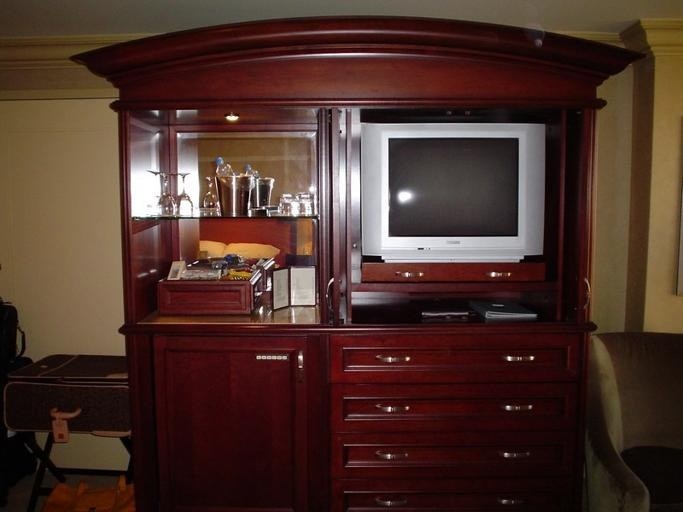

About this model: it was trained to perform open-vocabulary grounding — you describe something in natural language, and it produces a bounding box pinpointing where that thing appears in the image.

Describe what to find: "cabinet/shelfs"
[67,12,648,511]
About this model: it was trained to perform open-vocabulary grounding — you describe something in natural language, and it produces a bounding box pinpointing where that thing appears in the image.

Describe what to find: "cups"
[277,194,300,215]
[295,192,314,215]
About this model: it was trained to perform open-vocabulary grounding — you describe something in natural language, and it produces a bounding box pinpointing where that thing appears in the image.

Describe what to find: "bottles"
[244,164,261,178]
[216,157,236,177]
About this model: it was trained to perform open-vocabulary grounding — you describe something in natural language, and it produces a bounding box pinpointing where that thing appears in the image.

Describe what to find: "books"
[408,299,468,317]
[467,299,537,319]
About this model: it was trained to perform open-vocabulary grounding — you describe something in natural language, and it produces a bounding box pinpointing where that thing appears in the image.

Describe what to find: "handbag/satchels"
[43,485,136,511]
[1,303,17,364]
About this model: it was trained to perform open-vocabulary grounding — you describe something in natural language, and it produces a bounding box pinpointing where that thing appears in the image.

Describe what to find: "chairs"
[590,330,683,511]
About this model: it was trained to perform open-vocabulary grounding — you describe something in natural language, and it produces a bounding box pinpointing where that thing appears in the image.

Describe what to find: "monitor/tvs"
[360,123,546,264]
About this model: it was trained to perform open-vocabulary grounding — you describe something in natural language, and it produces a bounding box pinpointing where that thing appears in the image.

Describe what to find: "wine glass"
[202,176,219,209]
[174,170,194,217]
[158,170,177,217]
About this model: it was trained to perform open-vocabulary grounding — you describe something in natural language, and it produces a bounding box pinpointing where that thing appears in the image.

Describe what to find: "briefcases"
[3,353,132,437]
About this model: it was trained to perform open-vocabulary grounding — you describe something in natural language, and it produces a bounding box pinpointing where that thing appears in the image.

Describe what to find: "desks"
[11,430,131,512]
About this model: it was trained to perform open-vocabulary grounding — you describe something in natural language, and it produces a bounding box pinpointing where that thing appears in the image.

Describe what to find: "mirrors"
[176,133,318,305]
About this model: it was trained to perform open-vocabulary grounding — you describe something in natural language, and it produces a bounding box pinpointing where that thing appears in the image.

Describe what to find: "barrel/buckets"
[213,175,254,218]
[250,177,275,209]
